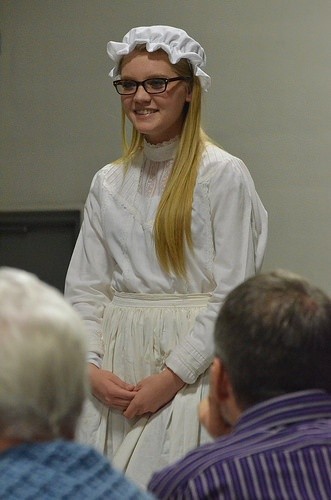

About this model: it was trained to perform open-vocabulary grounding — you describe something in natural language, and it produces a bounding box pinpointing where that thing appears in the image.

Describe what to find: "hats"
[106,25,212,92]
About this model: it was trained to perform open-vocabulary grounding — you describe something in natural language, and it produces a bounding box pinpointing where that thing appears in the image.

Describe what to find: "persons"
[1,264,162,500]
[61,23,270,497]
[146,270,331,500]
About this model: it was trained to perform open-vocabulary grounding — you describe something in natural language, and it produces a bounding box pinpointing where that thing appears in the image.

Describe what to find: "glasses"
[113,76,183,95]
